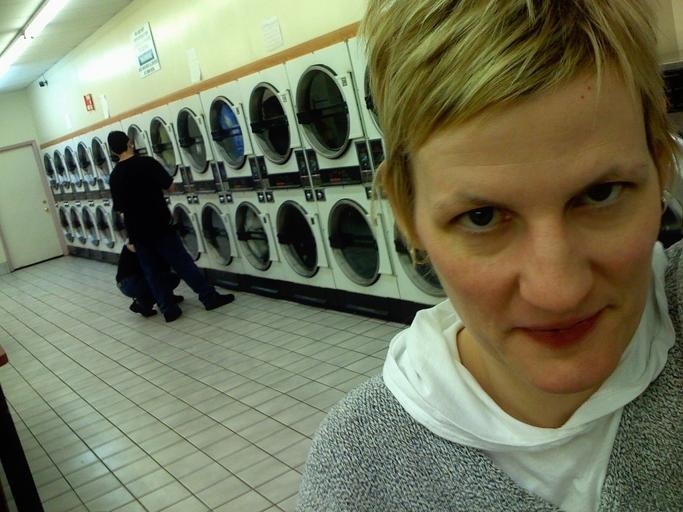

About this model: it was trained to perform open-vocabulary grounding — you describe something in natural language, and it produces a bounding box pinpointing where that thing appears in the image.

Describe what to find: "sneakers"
[130,300,157,317]
[164,308,182,322]
[173,296,184,304]
[203,293,235,311]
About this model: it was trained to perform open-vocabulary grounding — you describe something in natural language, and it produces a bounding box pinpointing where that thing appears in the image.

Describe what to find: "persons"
[117,235,183,317]
[295,0,682,512]
[108,130,236,322]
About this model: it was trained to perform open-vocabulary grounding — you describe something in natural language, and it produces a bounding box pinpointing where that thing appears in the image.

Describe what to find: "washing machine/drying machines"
[655,49,683,251]
[41,34,449,308]
